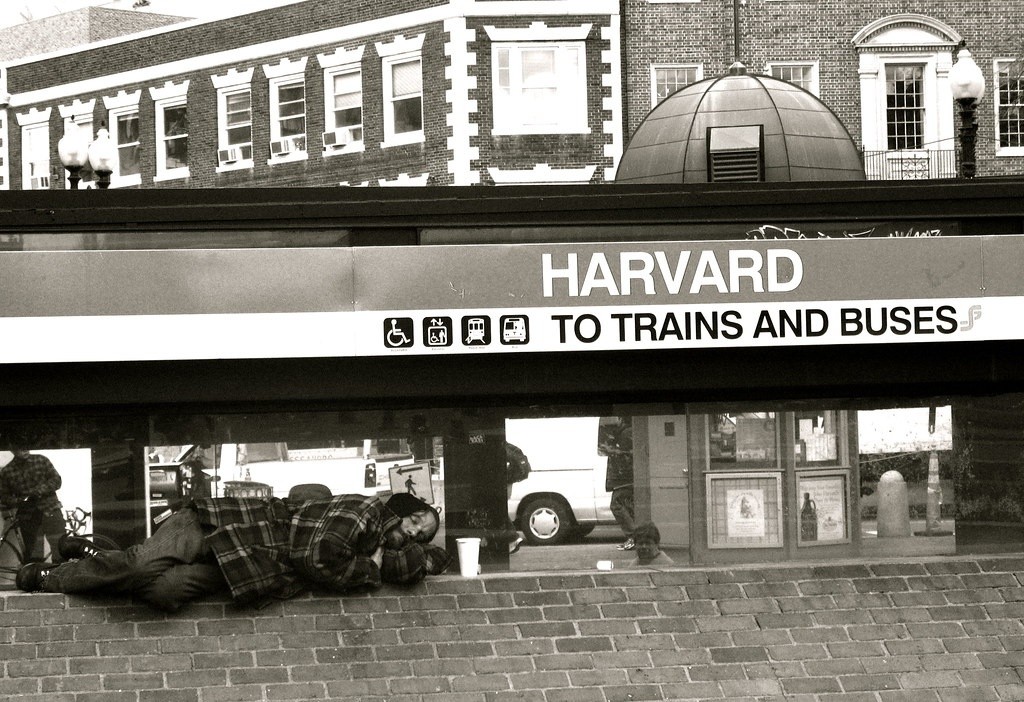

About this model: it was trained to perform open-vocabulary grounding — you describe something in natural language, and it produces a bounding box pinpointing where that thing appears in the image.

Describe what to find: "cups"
[455,538,481,577]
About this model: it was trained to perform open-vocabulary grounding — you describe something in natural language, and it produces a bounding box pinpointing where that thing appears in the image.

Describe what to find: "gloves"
[16,491,38,507]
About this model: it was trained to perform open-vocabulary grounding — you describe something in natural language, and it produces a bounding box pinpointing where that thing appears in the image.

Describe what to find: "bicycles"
[0,491,123,592]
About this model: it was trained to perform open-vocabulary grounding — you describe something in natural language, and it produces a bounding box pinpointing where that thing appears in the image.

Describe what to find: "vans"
[171,415,620,546]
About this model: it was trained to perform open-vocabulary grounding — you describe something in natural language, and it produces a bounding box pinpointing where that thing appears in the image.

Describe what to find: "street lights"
[948,46,985,178]
[58,118,116,192]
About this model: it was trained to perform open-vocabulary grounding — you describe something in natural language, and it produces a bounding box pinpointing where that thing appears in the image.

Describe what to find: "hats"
[386,492,431,518]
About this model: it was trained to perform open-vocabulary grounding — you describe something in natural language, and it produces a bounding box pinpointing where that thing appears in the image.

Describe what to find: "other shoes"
[617,538,636,551]
[509,537,523,554]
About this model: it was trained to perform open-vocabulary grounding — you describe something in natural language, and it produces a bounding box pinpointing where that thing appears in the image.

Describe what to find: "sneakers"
[16,562,61,593]
[58,537,123,562]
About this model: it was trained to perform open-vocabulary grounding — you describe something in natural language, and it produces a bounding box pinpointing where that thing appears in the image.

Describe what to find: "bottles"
[800,493,818,542]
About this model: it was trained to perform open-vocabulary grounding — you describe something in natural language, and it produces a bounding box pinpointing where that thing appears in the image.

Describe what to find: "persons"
[15,491,441,611]
[598,416,633,551]
[1,449,67,565]
[506,483,523,554]
[628,523,673,569]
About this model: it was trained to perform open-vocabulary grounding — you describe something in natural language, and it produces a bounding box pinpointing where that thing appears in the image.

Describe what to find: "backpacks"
[505,442,531,484]
